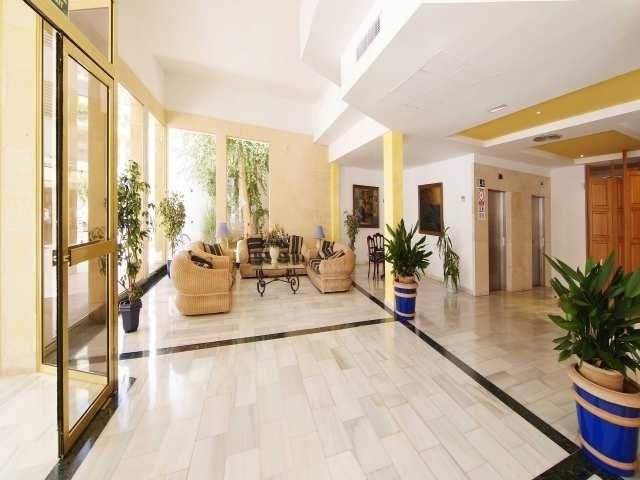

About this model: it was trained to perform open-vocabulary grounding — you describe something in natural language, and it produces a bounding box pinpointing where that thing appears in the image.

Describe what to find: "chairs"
[367,232,385,280]
[169,240,236,316]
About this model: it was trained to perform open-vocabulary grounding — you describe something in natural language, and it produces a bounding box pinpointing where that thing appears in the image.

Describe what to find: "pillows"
[247,235,303,254]
[319,239,345,260]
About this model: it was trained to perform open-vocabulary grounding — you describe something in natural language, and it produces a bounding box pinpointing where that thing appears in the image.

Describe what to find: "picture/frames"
[352,183,381,229]
[418,182,444,236]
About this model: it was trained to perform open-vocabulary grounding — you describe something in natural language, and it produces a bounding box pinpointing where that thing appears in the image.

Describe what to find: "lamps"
[311,224,325,250]
[215,221,233,248]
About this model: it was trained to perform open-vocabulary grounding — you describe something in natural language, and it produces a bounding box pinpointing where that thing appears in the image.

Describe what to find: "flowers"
[261,228,290,248]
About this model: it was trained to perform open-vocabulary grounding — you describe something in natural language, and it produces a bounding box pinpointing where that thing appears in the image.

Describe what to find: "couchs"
[237,234,308,278]
[307,242,355,294]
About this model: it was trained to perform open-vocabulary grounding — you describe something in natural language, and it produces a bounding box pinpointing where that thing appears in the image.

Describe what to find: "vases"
[269,246,280,259]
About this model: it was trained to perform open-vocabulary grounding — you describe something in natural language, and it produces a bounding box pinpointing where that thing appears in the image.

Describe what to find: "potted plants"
[541,250,639,476]
[155,192,187,278]
[378,218,433,320]
[436,224,462,290]
[88,159,155,333]
